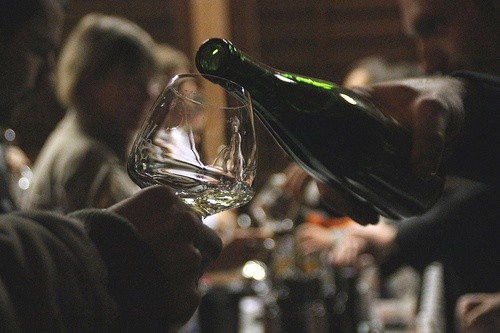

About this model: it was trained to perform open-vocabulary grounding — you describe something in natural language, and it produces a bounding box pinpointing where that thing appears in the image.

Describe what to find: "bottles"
[195,35,448,221]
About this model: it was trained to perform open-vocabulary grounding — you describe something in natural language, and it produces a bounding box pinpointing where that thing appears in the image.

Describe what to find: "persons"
[32,12,271,272]
[277,0,499,208]
[0,0,65,145]
[321,69,500,273]
[1,186,221,333]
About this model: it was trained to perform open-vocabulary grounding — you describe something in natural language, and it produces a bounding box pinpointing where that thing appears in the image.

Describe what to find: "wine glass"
[126,72,259,222]
